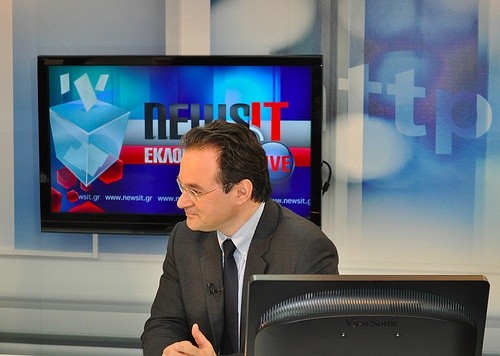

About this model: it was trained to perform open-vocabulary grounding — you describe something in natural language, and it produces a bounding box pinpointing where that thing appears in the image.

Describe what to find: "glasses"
[175,177,232,200]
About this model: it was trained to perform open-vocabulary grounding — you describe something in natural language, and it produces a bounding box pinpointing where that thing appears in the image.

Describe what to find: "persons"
[141,119,340,356]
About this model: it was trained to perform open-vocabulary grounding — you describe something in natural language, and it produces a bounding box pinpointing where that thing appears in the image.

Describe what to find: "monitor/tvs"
[244,275,490,356]
[37,54,322,236]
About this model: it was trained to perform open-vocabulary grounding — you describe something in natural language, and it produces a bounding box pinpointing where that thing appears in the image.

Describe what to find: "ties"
[219,238,239,355]
